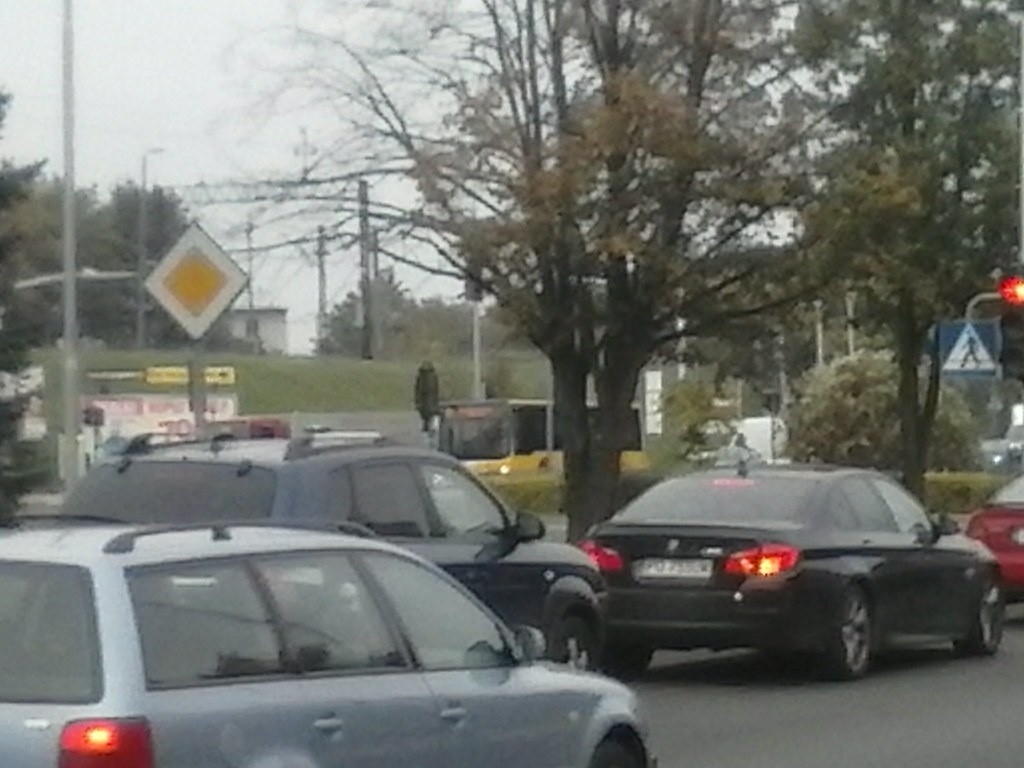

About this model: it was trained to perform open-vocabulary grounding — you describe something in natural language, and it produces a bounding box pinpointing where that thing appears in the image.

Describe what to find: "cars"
[577,460,1005,680]
[686,415,793,465]
[964,475,1024,603]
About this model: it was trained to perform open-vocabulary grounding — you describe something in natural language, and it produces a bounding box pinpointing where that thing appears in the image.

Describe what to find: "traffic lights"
[998,275,1024,306]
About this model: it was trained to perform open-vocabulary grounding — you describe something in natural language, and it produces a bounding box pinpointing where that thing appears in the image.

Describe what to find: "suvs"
[1,512,658,768]
[53,420,611,676]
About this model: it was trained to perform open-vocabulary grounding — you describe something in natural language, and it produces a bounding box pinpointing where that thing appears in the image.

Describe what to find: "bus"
[425,399,653,520]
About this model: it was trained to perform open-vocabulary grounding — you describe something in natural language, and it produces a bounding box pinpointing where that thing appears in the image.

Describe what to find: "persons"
[414,360,438,434]
